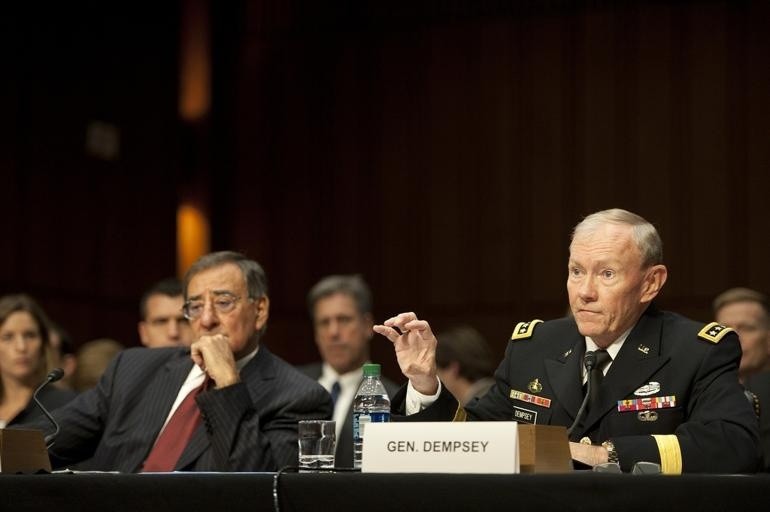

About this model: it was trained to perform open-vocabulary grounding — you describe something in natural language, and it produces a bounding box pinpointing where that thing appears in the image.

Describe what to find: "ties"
[582,350,612,404]
[331,382,341,405]
[142,367,216,471]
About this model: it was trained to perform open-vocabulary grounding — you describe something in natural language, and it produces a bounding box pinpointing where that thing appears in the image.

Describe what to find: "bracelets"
[601,439,618,464]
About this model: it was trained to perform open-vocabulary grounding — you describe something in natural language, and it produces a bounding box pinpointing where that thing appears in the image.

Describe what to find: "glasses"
[180,294,252,321]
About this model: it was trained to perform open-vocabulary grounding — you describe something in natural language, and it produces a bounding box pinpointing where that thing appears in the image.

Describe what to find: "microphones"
[518,351,597,474]
[0,367,64,475]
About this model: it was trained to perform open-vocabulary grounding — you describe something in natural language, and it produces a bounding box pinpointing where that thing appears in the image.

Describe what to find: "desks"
[1,472,769,511]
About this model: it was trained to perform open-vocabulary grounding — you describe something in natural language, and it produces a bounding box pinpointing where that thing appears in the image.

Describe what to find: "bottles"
[352,363,391,473]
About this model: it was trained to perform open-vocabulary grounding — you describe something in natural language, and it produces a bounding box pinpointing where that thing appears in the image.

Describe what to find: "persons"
[373,207,763,475]
[713,286,770,472]
[295,274,401,467]
[0,295,79,429]
[436,316,505,403]
[45,276,197,392]
[9,250,333,470]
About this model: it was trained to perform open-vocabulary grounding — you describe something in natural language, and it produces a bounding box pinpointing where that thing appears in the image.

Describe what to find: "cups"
[298,419,336,473]
